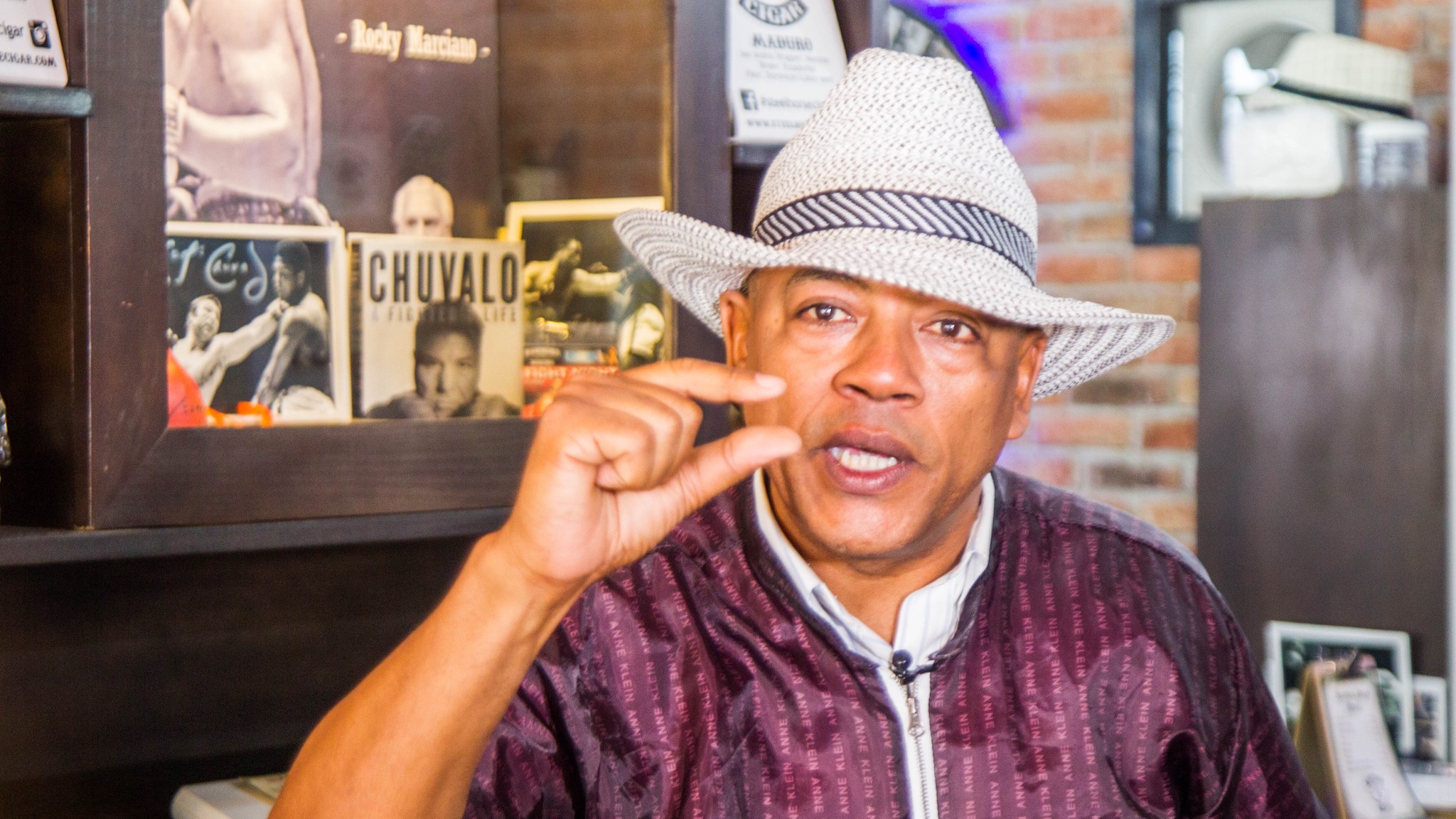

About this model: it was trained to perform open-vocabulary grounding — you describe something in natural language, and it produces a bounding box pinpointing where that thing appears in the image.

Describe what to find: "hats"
[1247,26,1433,126]
[614,46,1175,399]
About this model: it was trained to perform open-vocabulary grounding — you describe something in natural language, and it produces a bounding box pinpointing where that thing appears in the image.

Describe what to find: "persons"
[163,1,320,222]
[368,300,516,417]
[166,241,328,418]
[392,175,453,237]
[522,236,665,369]
[267,48,1327,819]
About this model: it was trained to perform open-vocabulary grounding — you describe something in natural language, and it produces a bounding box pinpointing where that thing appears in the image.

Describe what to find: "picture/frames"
[1128,1,1366,248]
[1266,620,1448,755]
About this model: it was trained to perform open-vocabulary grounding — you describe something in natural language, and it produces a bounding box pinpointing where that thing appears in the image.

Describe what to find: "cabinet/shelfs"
[0,0,879,568]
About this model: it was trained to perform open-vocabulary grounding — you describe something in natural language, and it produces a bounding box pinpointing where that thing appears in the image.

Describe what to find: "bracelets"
[167,89,183,142]
